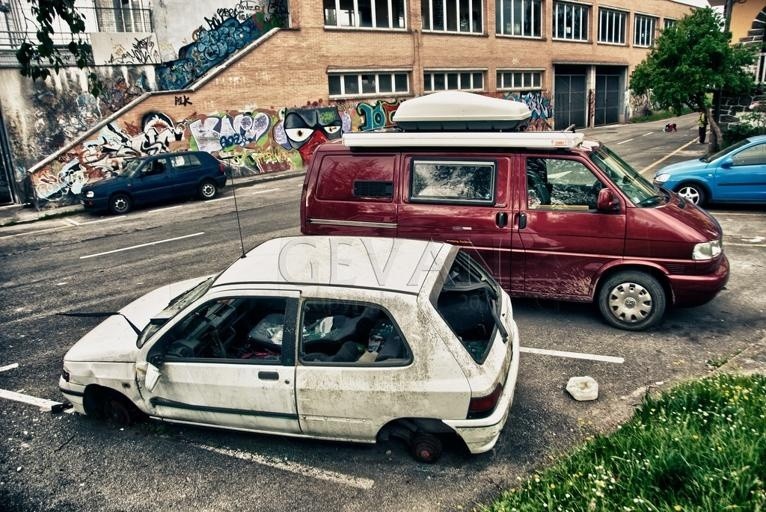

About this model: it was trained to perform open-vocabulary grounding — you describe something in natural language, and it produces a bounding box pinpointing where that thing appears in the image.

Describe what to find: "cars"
[652,136,766,209]
[81,149,228,214]
[58,232,519,466]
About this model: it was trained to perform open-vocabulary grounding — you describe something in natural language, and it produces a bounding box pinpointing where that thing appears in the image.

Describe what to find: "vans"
[299,88,731,331]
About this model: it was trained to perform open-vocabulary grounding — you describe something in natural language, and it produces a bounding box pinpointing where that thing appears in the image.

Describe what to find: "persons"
[698,113,708,144]
